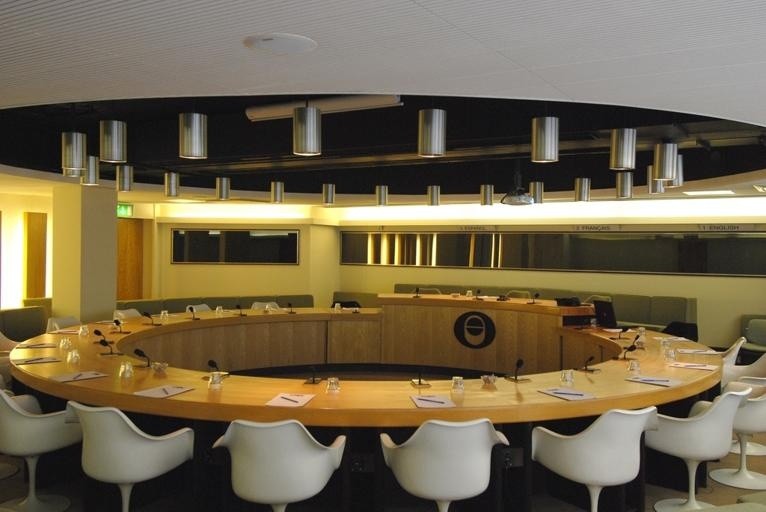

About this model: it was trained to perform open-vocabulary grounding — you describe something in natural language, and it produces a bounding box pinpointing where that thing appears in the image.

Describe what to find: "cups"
[628,327,677,377]
[326,377,340,394]
[60,301,345,367]
[207,371,223,391]
[450,377,465,393]
[560,369,574,384]
[119,360,135,378]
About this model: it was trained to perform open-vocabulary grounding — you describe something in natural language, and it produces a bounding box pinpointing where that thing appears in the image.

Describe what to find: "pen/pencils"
[57,331,77,334]
[73,373,82,379]
[642,380,669,382]
[30,344,44,346]
[163,388,168,395]
[553,392,583,396]
[418,398,445,404]
[693,350,707,353]
[281,396,298,403]
[685,365,707,367]
[25,358,44,362]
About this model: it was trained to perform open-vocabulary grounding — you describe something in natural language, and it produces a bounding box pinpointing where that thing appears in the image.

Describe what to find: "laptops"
[594,299,641,330]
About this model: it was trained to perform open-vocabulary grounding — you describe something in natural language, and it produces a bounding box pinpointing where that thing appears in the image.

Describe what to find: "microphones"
[574,355,599,373]
[473,289,484,300]
[133,350,153,369]
[505,359,530,382]
[304,366,320,384]
[287,303,295,315]
[609,328,645,360]
[412,288,422,298]
[526,292,540,304]
[412,368,432,388]
[141,311,161,327]
[188,306,200,321]
[203,359,228,384]
[235,304,248,316]
[93,318,131,355]
[352,302,363,313]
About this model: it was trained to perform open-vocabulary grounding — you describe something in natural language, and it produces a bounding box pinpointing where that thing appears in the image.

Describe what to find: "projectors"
[500,194,535,204]
